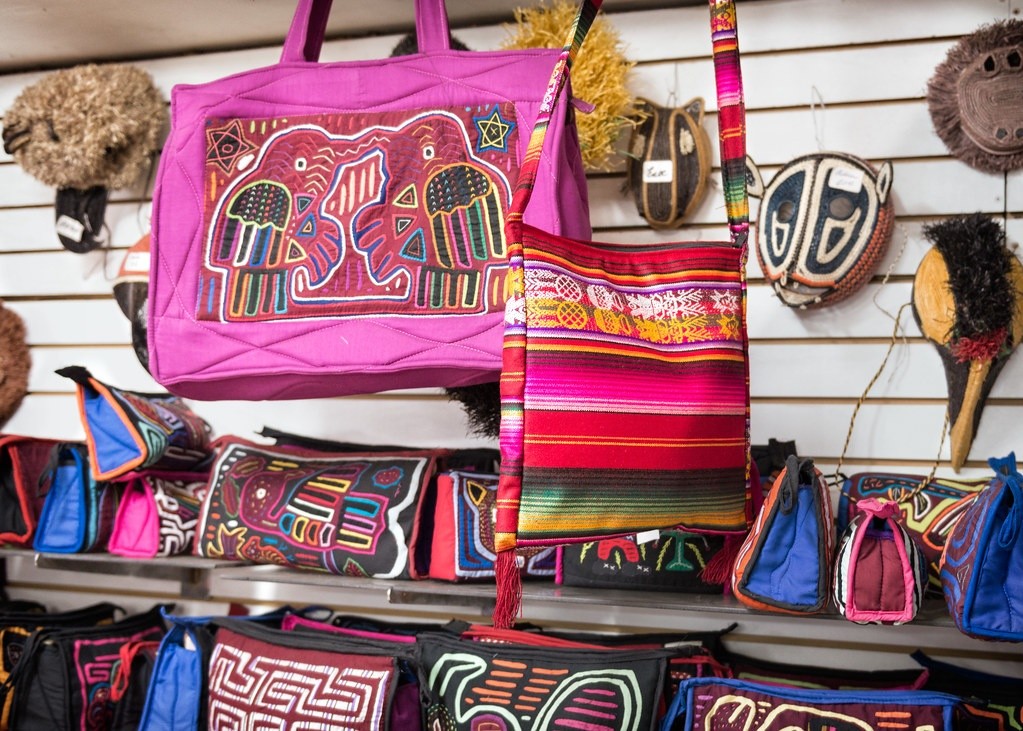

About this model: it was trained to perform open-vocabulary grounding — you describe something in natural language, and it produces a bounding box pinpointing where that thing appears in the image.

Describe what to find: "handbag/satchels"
[494,0,751,634]
[0,367,1023,731]
[147,0,592,400]
